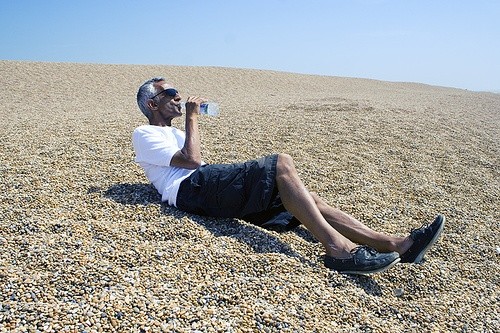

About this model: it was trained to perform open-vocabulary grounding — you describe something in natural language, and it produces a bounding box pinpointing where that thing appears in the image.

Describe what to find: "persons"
[130,75,446,275]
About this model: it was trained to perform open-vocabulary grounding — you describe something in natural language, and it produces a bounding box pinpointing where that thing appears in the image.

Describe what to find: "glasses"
[151,88,178,99]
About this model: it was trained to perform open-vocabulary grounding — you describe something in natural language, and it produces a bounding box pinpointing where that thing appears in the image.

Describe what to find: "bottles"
[180,101,219,115]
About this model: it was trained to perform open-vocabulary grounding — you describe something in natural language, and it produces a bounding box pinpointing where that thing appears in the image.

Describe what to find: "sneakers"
[324,243,400,275]
[399,214,446,264]
